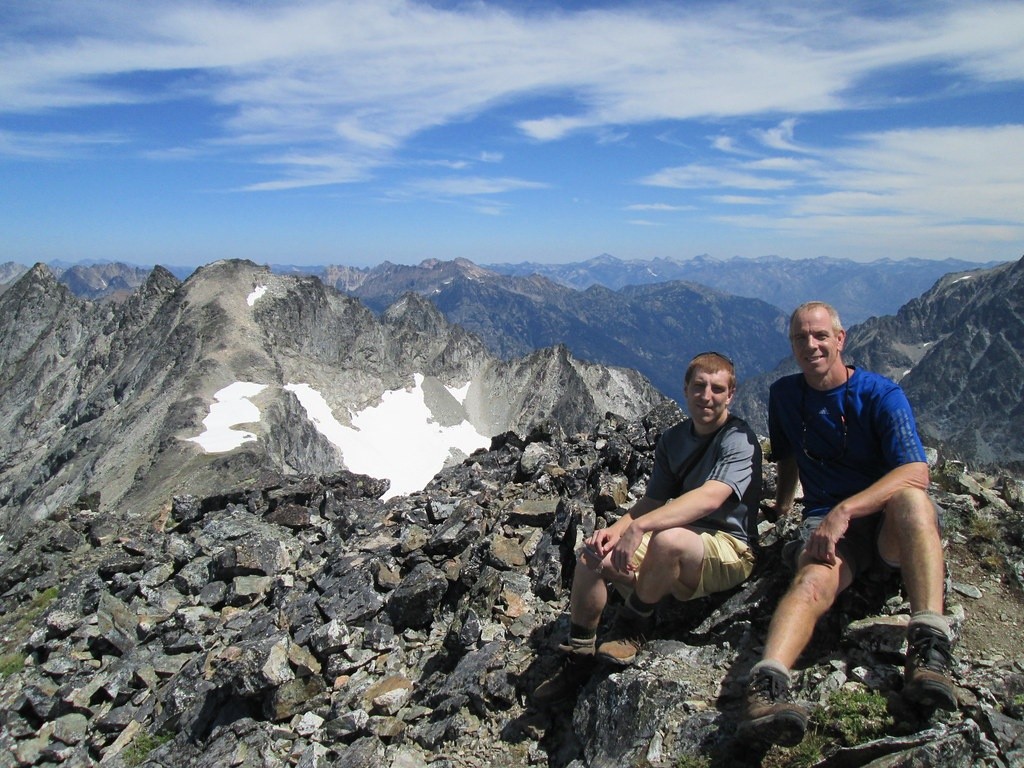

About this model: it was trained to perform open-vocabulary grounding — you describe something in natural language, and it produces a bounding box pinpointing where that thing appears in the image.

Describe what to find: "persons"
[530,352,762,700]
[735,301,957,748]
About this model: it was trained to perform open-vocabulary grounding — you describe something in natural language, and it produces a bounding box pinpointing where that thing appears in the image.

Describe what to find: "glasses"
[799,421,848,466]
[692,351,734,367]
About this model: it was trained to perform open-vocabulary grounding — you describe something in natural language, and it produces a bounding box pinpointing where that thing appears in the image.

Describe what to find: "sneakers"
[532,643,597,709]
[902,628,958,713]
[598,601,658,665]
[733,665,807,753]
